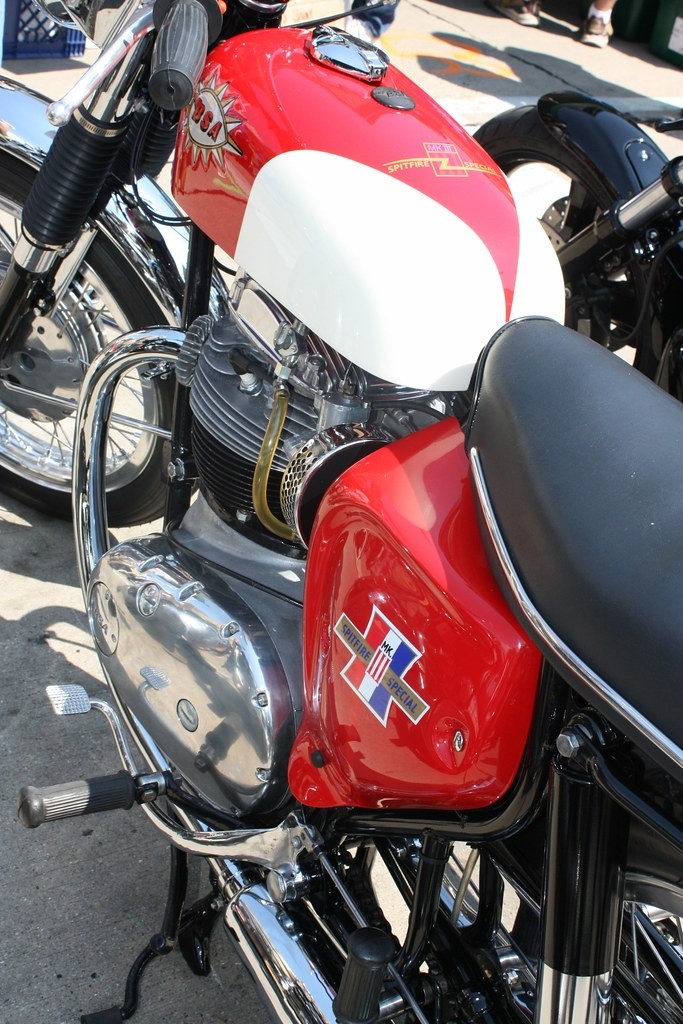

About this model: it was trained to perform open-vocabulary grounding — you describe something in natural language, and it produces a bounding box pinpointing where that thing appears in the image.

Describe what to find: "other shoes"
[580,15,614,48]
[484,0,541,26]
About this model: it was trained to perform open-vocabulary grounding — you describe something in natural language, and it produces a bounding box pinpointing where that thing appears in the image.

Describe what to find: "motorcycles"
[0,1,682,1024]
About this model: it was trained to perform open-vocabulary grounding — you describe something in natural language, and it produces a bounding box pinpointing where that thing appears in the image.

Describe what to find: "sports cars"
[466,91,683,398]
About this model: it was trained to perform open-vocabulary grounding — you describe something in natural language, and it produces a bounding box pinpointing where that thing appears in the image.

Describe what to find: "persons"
[483,0,618,48]
